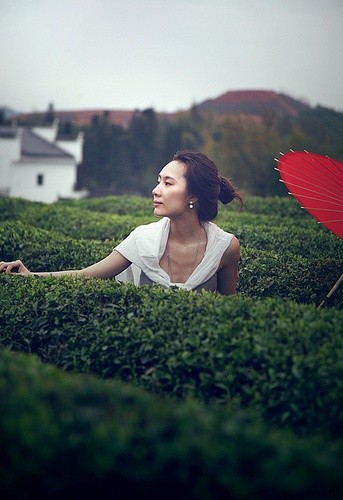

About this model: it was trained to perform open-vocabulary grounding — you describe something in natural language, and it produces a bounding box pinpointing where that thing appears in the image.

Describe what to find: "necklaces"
[165,224,202,285]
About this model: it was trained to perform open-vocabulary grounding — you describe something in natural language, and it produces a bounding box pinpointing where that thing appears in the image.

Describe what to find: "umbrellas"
[273,149,343,310]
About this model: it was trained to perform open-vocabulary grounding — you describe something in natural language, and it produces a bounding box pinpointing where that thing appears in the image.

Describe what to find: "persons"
[0,151,243,296]
